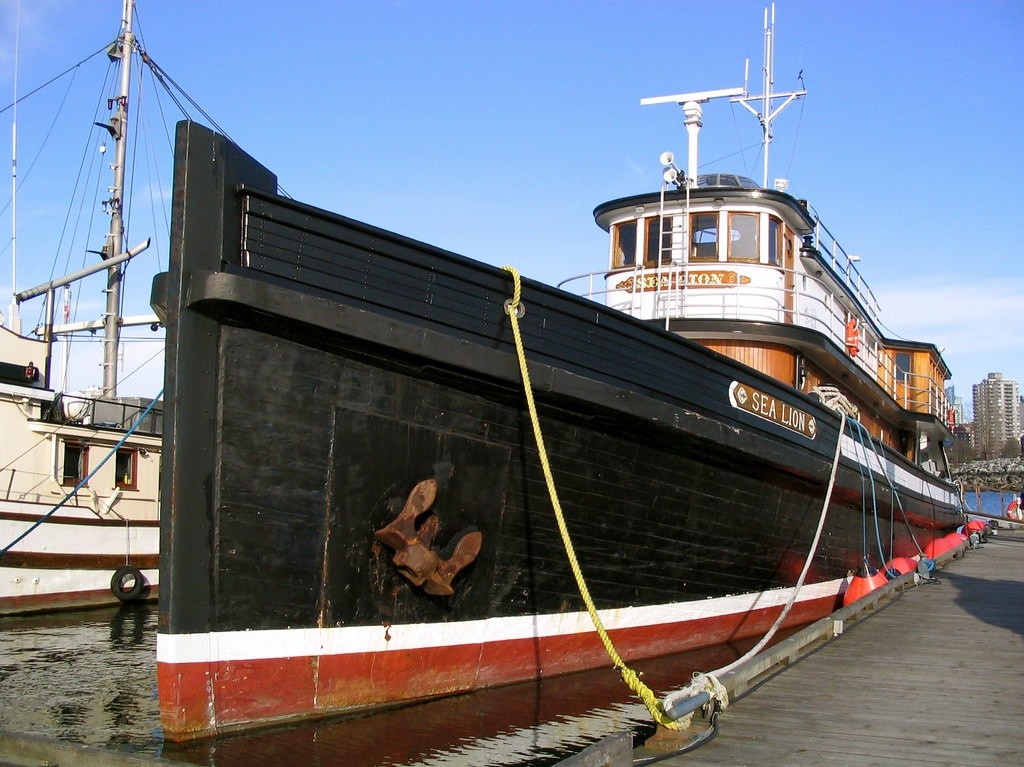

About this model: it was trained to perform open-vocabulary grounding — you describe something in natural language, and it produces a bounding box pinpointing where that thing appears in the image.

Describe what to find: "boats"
[148,1,969,744]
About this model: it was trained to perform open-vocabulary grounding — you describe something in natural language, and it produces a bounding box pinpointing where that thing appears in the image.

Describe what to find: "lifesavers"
[846,318,860,358]
[110,565,144,602]
[1007,500,1024,522]
[950,410,955,430]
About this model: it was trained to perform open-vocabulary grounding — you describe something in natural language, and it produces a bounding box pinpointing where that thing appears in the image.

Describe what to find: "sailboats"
[0,0,301,618]
